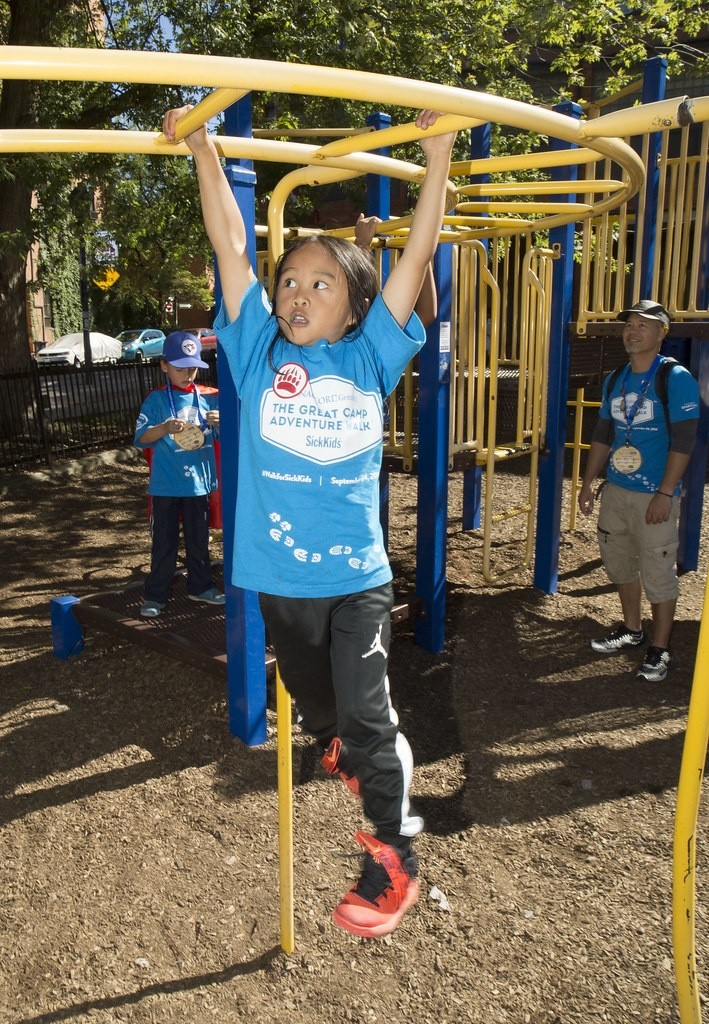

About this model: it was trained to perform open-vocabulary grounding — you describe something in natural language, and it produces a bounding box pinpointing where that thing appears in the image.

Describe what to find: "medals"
[174,425,204,449]
[612,446,642,473]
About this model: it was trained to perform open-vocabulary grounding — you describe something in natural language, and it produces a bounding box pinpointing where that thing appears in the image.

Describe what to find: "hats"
[617,299,670,331]
[162,330,209,368]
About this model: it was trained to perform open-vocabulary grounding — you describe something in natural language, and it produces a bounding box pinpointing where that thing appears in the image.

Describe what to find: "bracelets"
[657,490,673,498]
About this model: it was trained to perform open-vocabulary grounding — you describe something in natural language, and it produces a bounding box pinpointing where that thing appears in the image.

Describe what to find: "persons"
[577,300,700,683]
[134,331,226,617]
[162,103,458,935]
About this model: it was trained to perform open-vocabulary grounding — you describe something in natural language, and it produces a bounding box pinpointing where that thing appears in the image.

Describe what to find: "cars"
[115,329,167,363]
[183,328,217,350]
[35,332,122,369]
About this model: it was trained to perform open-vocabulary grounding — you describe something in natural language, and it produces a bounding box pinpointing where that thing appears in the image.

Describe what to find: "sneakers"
[589,628,645,652]
[334,831,420,938]
[636,649,673,683]
[141,599,166,617]
[321,736,363,801]
[189,587,226,604]
